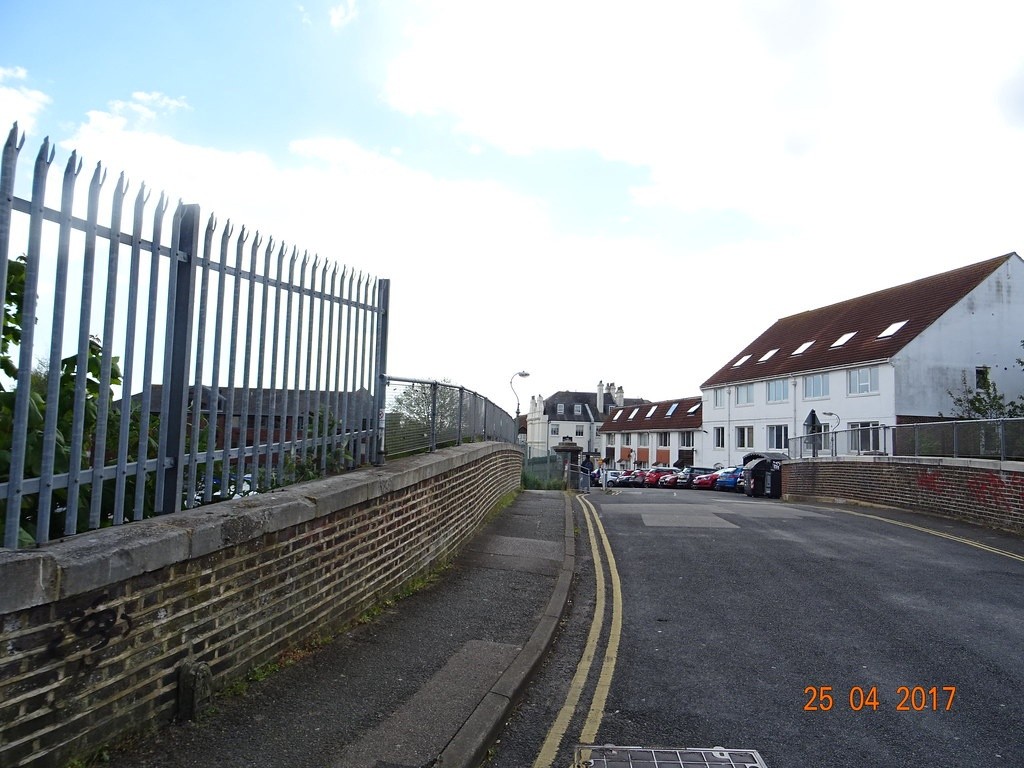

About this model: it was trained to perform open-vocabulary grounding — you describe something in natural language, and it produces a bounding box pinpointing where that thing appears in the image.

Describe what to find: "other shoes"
[587,491,590,494]
[582,490,584,493]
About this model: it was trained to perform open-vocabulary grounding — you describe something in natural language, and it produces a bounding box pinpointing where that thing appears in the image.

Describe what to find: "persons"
[581,454,594,494]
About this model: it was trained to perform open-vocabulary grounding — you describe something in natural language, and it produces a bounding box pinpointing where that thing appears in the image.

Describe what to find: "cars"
[717,466,743,493]
[658,472,682,489]
[692,466,736,490]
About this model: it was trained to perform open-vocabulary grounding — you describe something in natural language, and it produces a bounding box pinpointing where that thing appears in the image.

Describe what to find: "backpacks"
[584,460,593,473]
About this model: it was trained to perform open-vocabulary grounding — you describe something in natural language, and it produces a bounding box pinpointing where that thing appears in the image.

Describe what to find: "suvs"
[676,466,716,489]
[590,467,649,487]
[645,466,679,488]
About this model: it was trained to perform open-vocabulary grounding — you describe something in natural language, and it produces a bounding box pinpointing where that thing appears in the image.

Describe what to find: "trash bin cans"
[742,453,791,499]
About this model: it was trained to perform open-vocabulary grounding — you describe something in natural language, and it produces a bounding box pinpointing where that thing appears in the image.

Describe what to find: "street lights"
[824,412,840,456]
[509,371,530,442]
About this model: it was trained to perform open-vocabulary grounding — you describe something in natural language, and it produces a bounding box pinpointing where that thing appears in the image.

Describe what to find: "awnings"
[602,458,610,464]
[673,459,687,467]
[634,461,641,464]
[617,459,623,463]
[652,462,660,466]
